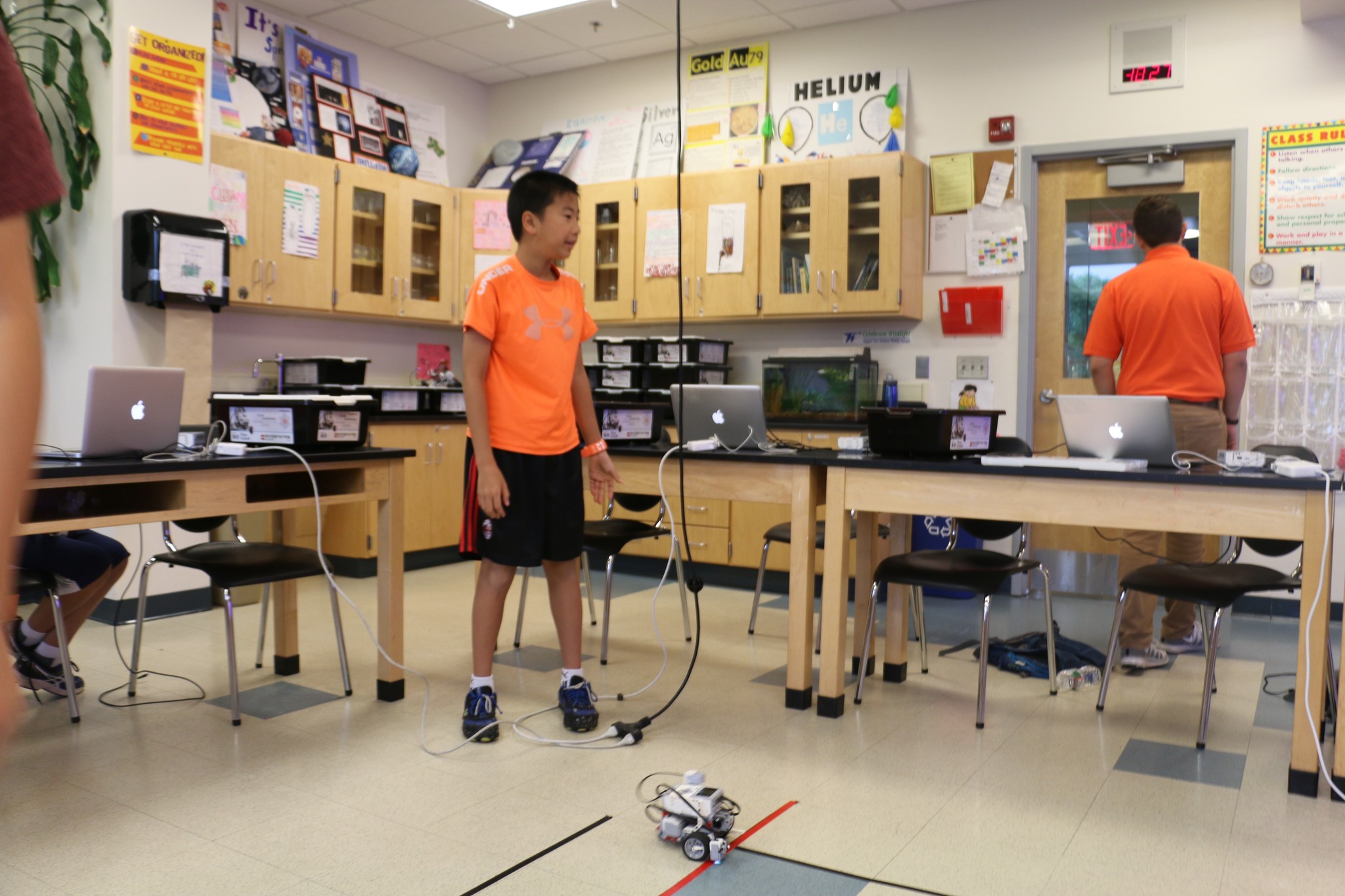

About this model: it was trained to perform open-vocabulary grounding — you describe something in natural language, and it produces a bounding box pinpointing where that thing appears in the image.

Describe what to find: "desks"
[11,447,417,702]
[816,448,1345,799]
[581,441,877,712]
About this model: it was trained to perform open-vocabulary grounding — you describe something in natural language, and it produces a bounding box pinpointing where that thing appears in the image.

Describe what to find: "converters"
[1275,460,1322,477]
[687,439,718,452]
[213,442,247,456]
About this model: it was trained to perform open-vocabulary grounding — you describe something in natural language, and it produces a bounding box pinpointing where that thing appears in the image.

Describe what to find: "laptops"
[670,384,775,450]
[1056,395,1205,467]
[36,366,187,459]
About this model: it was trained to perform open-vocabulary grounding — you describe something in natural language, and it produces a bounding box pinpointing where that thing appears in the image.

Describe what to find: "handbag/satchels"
[972,631,1108,679]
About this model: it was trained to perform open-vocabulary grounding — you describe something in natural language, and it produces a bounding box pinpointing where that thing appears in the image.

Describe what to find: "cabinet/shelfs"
[334,160,457,329]
[604,425,734,587]
[729,426,913,603]
[634,165,762,328]
[280,422,469,581]
[760,149,929,325]
[440,187,519,334]
[551,178,636,328]
[209,132,338,319]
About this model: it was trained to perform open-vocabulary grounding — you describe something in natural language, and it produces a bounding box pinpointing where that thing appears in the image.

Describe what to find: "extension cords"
[612,716,652,742]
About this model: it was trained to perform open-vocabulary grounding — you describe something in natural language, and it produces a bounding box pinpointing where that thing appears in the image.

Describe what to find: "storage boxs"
[283,356,372,384]
[648,337,735,365]
[589,362,648,389]
[326,386,430,422]
[591,336,648,363]
[761,355,880,432]
[647,363,735,390]
[593,388,643,403]
[424,386,468,421]
[859,405,1008,458]
[206,392,377,454]
[645,389,672,402]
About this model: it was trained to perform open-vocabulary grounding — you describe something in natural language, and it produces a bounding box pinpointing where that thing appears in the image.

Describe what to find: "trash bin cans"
[911,515,984,600]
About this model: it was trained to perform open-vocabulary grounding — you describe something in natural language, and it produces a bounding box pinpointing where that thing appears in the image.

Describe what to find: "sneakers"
[12,652,84,696]
[6,617,36,658]
[558,675,598,732]
[462,686,499,742]
[1120,645,1169,669]
[1160,621,1219,653]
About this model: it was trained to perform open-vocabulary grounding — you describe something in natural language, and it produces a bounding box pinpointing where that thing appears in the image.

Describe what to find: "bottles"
[882,372,899,409]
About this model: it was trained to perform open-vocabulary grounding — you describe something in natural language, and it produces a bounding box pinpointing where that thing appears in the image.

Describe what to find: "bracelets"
[579,438,608,458]
[1227,418,1239,425]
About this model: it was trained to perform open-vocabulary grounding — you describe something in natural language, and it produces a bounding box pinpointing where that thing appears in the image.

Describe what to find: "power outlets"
[956,355,989,380]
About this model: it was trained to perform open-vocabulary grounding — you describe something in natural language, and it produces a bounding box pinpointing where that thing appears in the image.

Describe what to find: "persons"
[0,25,131,747]
[461,169,624,746]
[1084,194,1255,671]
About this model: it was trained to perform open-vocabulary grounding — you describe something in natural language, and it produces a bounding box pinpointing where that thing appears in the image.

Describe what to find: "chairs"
[127,425,353,728]
[854,434,1058,730]
[748,507,889,657]
[513,426,694,667]
[9,569,83,725]
[1095,444,1339,751]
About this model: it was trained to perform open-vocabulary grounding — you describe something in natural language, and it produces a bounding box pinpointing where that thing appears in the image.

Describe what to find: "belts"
[1171,395,1217,408]
